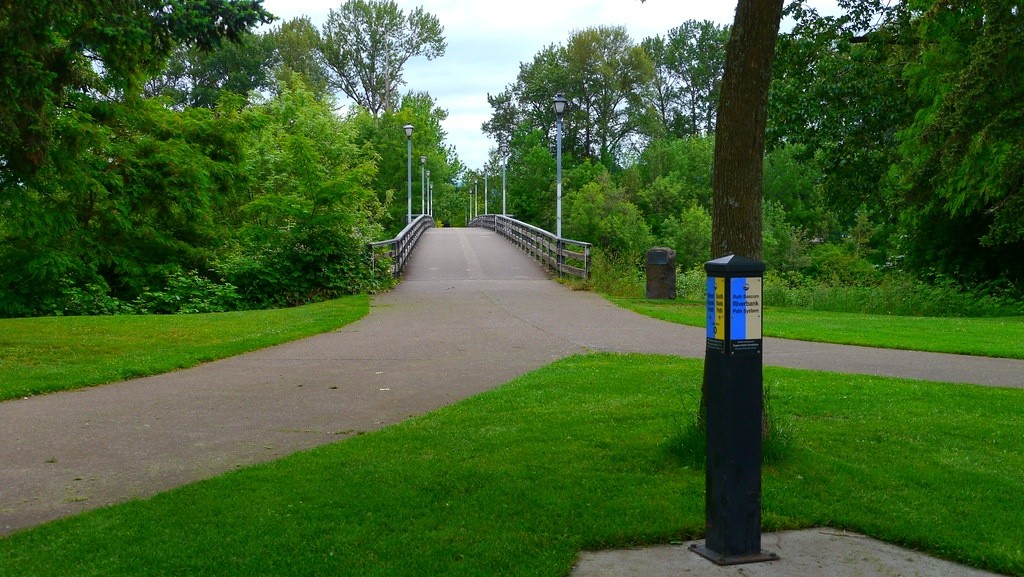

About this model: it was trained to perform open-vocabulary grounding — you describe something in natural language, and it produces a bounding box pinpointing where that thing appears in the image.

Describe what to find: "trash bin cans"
[645,247,678,299]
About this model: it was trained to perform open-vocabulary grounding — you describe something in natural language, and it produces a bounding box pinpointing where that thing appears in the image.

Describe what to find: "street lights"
[501,143,509,216]
[469,190,472,221]
[420,155,425,214]
[403,122,414,224]
[426,171,430,215]
[551,92,569,238]
[430,181,433,216]
[474,180,478,217]
[483,162,489,214]
